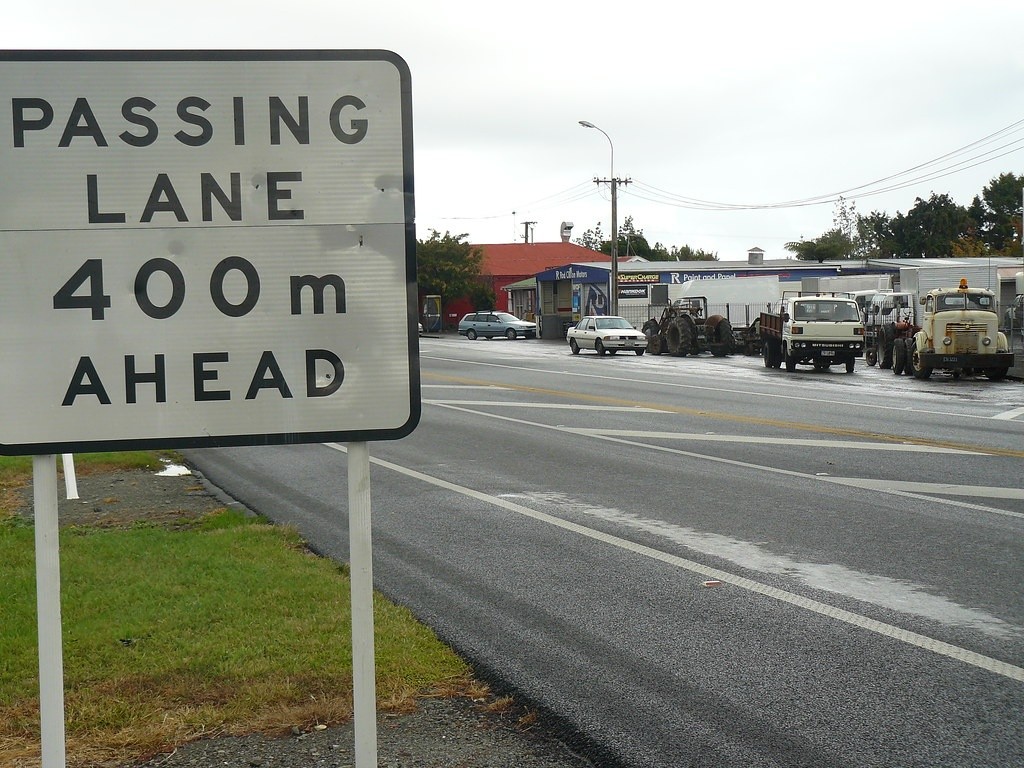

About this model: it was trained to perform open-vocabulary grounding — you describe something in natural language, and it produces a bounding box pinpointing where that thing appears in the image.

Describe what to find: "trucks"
[759,290,865,372]
[801,265,998,345]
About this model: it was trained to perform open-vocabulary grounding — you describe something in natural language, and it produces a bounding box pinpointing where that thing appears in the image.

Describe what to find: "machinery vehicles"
[876,306,922,374]
[642,296,735,357]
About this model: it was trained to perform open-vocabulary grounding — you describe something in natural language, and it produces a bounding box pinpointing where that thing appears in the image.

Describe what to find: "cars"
[418,323,424,336]
[566,315,649,357]
[908,278,1015,381]
[457,312,539,340]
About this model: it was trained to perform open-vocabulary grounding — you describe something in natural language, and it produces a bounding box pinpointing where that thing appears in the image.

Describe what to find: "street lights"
[512,212,516,244]
[578,119,620,316]
